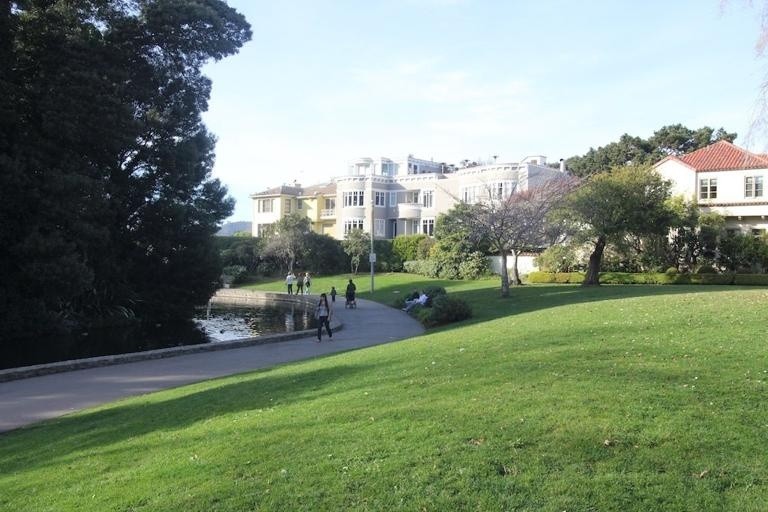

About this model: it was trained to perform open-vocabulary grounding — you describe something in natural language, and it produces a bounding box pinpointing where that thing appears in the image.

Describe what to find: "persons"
[285,271,356,309]
[315,293,334,343]
[402,288,429,312]
